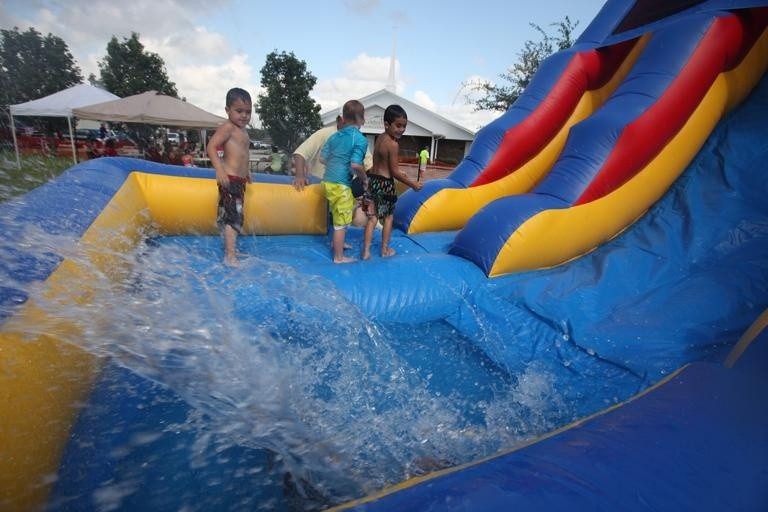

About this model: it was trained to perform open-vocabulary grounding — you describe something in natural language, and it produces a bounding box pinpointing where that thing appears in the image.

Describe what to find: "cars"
[167,133,180,147]
[250,141,271,150]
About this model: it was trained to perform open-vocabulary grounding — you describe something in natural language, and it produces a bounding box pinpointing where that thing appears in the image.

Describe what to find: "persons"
[207,88,252,268]
[37,122,212,169]
[264,143,282,175]
[416,144,430,183]
[359,104,422,261]
[291,106,408,226]
[318,99,369,265]
